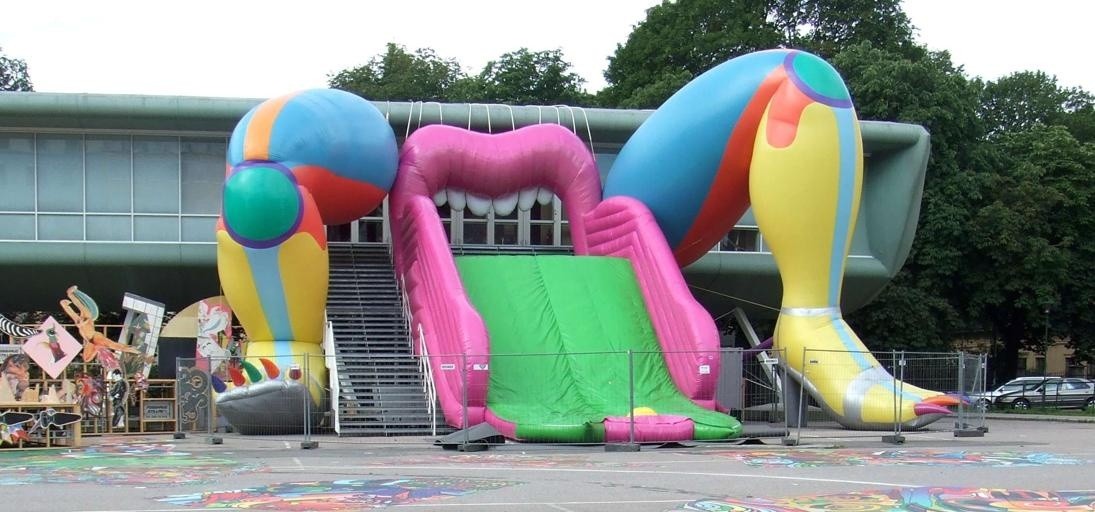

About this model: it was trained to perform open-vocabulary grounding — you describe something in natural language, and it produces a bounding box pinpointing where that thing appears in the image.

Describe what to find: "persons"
[109,370,128,426]
[59,285,141,363]
[976,335,1005,391]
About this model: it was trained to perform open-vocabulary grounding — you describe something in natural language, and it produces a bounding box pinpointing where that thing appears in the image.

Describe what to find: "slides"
[454,256,743,440]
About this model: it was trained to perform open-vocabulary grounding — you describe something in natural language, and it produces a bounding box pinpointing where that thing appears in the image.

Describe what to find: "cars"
[967,376,1095,414]
